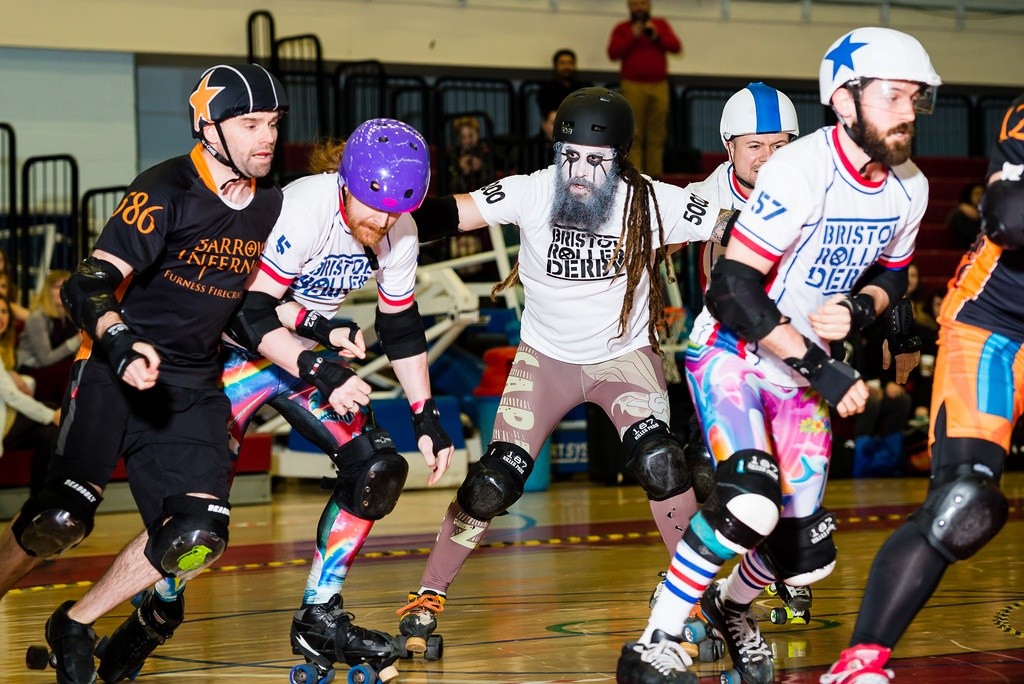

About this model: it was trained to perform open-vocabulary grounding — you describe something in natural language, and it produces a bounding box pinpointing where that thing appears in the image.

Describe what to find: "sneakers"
[819,644,895,684]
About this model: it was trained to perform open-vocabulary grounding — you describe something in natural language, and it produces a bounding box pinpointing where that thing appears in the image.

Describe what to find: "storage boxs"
[286,391,471,491]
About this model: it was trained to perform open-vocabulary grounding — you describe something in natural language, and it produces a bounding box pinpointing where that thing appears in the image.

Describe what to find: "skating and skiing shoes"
[26,599,101,684]
[94,589,185,684]
[290,593,402,684]
[616,629,698,684]
[765,576,813,625]
[396,590,447,659]
[684,578,775,684]
[648,571,715,643]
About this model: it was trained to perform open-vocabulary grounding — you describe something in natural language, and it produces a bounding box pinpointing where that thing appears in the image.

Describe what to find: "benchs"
[279,148,995,302]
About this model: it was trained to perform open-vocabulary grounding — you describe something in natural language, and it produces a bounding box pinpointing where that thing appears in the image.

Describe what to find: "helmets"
[818,27,941,104]
[188,63,290,138]
[338,117,431,212]
[720,82,800,146]
[553,86,635,160]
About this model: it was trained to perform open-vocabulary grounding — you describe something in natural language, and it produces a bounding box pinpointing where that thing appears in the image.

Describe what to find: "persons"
[1,62,365,684]
[901,262,947,416]
[396,81,813,684]
[45,118,455,684]
[0,253,83,500]
[614,28,943,684]
[441,0,682,189]
[818,92,1024,684]
[947,182,983,249]
